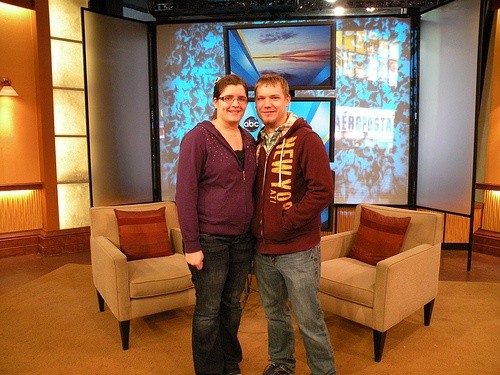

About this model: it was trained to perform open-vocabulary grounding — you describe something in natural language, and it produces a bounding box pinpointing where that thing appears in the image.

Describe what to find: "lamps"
[0,79,19,96]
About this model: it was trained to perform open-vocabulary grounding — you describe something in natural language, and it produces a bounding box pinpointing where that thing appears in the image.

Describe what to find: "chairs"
[89,201,196,351]
[318,203,443,363]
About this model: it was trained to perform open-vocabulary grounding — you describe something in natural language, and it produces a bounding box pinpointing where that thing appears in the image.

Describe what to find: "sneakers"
[263,362,290,375]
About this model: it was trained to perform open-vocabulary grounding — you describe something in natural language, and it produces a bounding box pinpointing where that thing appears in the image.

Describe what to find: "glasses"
[217,96,248,105]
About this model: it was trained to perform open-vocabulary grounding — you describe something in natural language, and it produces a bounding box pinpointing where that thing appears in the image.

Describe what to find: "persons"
[177,74,258,375]
[254,74,337,375]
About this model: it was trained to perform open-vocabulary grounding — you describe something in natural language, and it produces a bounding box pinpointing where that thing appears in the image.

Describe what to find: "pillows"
[347,205,411,266]
[113,207,173,262]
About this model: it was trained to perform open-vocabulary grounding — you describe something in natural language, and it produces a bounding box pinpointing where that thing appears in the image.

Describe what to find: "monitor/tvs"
[240,98,336,162]
[319,171,335,232]
[222,21,337,97]
